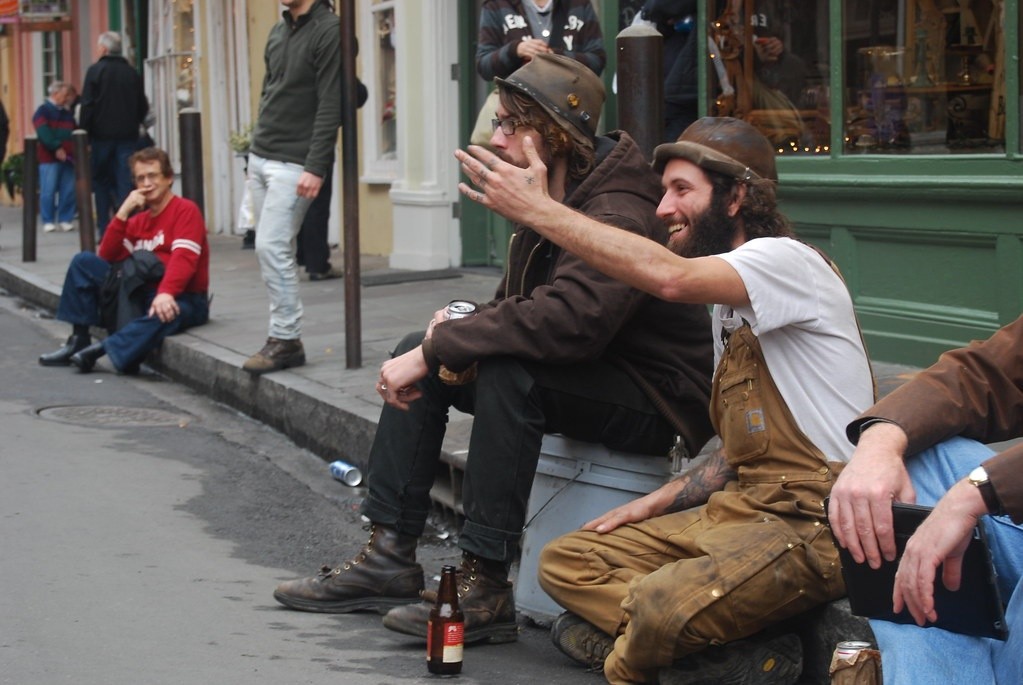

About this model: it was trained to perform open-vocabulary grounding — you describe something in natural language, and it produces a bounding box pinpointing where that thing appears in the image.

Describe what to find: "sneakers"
[658,630,803,685]
[550,612,616,673]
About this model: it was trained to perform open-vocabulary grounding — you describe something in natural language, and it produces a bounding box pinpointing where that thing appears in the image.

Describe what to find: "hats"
[494,53,605,152]
[650,116,779,194]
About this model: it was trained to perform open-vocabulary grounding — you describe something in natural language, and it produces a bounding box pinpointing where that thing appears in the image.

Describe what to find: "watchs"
[969,465,1001,516]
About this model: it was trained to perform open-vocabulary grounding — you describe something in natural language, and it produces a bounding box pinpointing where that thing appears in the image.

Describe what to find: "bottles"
[904,29,935,132]
[426,565,464,675]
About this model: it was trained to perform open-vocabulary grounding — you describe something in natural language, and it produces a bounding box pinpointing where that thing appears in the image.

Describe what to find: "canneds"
[328,461,362,486]
[445,301,476,320]
[836,641,873,660]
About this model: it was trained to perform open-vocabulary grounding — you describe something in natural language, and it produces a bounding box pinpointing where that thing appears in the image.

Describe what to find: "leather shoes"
[40,333,92,366]
[72,342,107,370]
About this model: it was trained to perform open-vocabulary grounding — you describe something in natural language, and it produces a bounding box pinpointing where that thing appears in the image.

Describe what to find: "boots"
[381,557,518,644]
[274,523,424,613]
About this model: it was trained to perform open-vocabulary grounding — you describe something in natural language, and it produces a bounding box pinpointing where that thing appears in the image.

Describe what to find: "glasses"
[492,120,529,135]
[135,172,162,182]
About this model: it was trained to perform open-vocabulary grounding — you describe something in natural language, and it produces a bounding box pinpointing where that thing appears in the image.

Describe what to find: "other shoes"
[300,263,343,280]
[243,338,305,373]
[43,222,54,231]
[58,222,74,231]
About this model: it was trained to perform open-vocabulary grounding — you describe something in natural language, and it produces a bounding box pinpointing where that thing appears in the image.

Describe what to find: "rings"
[430,319,435,324]
[382,385,387,392]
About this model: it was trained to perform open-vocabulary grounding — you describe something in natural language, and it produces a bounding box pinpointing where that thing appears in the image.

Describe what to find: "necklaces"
[532,9,551,37]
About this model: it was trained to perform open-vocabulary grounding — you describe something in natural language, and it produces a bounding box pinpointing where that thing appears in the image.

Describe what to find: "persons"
[32,81,80,233]
[273,51,714,644]
[39,147,209,376]
[453,117,875,685]
[235,0,368,375]
[642,0,697,147]
[476,0,606,81]
[0,100,10,182]
[79,31,149,245]
[4,165,16,201]
[828,313,1023,685]
[713,0,819,110]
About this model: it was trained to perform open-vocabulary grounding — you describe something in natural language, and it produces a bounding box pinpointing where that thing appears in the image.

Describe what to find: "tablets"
[823,496,1009,642]
